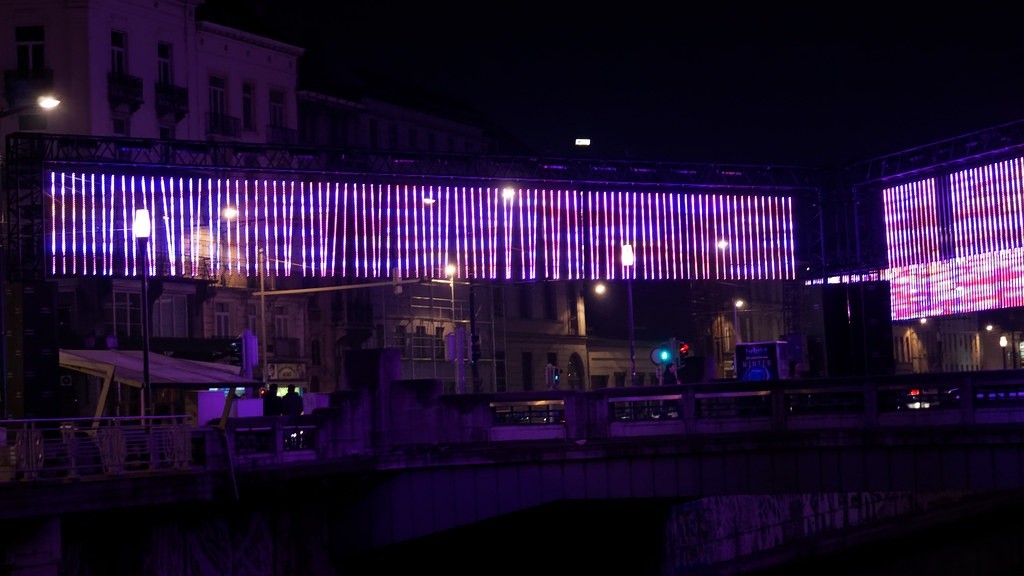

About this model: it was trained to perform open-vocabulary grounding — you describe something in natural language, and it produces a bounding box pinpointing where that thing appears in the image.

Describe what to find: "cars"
[632,386,961,422]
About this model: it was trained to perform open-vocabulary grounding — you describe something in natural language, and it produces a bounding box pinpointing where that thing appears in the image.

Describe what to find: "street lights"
[999,336,1008,369]
[133,208,151,437]
[620,244,638,421]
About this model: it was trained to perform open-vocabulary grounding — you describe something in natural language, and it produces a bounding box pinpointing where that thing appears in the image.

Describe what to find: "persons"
[262,383,304,415]
